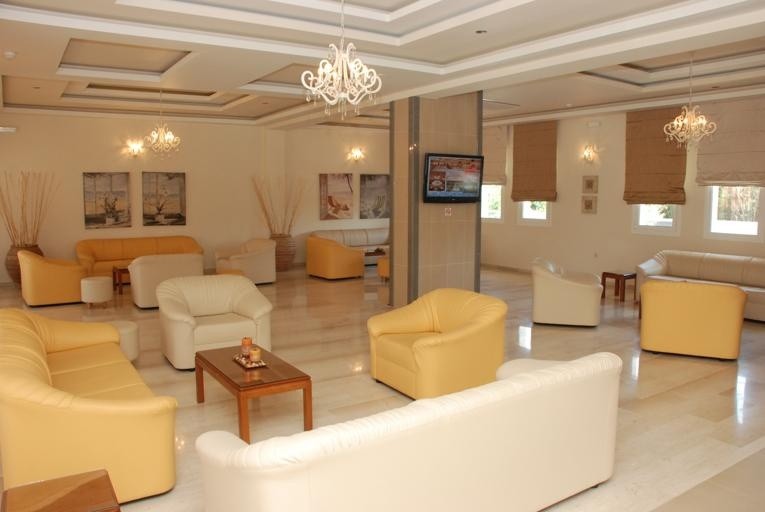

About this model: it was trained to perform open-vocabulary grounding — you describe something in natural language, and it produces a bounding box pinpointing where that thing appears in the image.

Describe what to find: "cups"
[249,348,261,362]
[242,337,252,356]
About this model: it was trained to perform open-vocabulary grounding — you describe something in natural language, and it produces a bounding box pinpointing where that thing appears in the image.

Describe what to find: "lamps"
[663,54,718,146]
[301,1,382,121]
[144,90,182,160]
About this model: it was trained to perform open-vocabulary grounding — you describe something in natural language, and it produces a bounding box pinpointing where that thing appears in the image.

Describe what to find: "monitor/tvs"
[423,152,484,203]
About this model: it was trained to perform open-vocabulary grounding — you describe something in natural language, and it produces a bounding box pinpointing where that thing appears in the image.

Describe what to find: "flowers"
[148,190,171,222]
[0,168,63,245]
[252,174,304,236]
[98,192,117,226]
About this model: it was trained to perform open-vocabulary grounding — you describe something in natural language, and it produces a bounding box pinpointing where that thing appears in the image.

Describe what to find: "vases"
[5,245,43,288]
[271,235,299,270]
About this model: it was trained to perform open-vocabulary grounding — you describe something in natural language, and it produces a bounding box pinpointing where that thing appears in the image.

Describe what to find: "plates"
[234,354,266,369]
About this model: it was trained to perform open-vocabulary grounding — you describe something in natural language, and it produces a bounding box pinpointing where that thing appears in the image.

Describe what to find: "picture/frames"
[360,174,390,219]
[141,172,187,226]
[319,173,354,221]
[580,195,598,215]
[582,176,599,194]
[83,171,133,229]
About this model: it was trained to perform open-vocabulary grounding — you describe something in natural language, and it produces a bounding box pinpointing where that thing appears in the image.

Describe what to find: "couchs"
[17,251,84,306]
[77,236,202,285]
[636,250,765,323]
[195,351,625,512]
[216,239,276,285]
[532,257,604,328]
[0,307,177,507]
[306,236,365,281]
[366,287,507,400]
[640,280,748,360]
[312,228,391,265]
[128,252,204,309]
[157,276,272,371]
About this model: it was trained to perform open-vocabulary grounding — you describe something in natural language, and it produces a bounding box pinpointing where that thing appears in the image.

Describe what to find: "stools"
[0,469,122,512]
[108,320,140,368]
[81,275,114,309]
[602,272,638,303]
